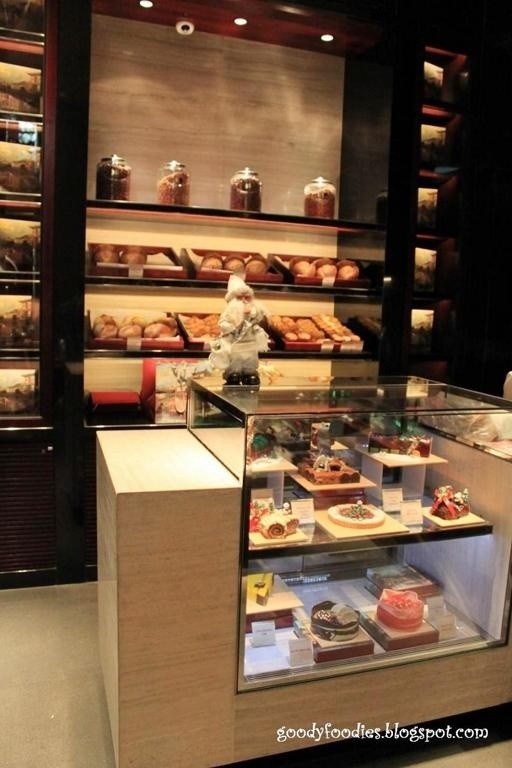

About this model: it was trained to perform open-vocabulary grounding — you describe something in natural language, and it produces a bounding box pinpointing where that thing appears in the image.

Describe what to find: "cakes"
[429,485,471,520]
[327,500,385,529]
[376,589,424,629]
[247,572,274,606]
[250,500,299,539]
[310,601,360,642]
[297,456,360,485]
[368,431,432,457]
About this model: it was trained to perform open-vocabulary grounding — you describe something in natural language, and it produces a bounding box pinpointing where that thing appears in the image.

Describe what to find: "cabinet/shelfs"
[0,1,512,591]
[98,376,512,768]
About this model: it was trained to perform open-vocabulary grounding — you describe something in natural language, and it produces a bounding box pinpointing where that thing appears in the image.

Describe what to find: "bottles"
[94,153,132,202]
[302,176,337,220]
[156,160,190,207]
[229,167,263,213]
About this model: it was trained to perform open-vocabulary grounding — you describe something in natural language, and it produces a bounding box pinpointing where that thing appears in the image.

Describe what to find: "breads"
[92,245,360,342]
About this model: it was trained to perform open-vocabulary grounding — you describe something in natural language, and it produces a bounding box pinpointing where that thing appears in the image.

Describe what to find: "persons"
[211,273,274,387]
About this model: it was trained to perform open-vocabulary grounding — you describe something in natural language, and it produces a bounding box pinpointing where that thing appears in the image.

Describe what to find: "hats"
[225,273,253,298]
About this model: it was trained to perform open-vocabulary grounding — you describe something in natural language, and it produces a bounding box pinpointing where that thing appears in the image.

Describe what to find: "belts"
[226,371,261,385]
